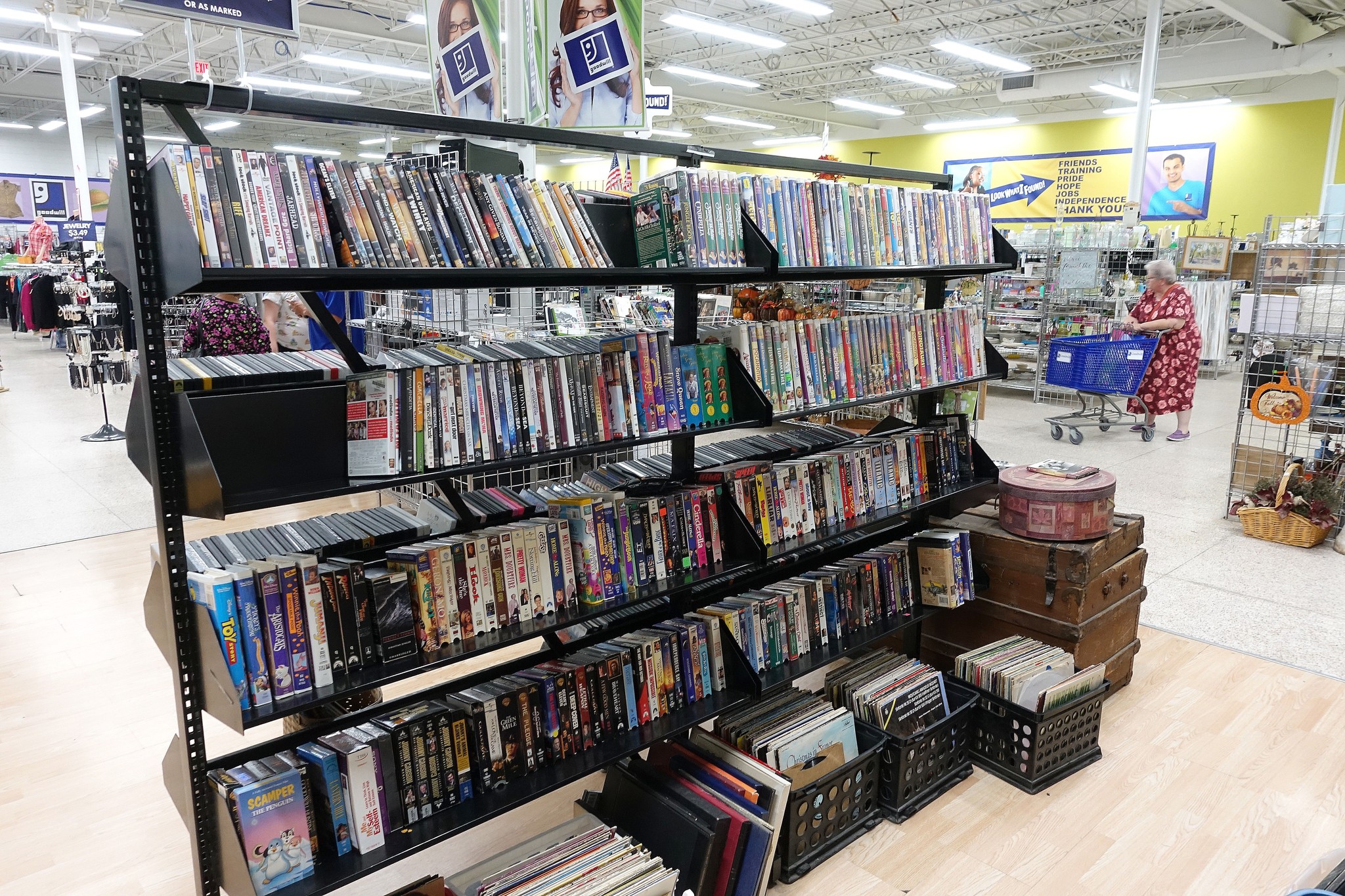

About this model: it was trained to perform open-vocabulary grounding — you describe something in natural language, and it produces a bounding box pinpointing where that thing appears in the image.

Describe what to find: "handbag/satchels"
[183,297,209,358]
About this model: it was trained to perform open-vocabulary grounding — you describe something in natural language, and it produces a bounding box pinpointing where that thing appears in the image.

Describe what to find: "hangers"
[0,266,62,285]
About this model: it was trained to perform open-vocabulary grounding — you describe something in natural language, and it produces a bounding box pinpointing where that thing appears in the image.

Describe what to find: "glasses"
[449,19,471,33]
[576,7,608,19]
[1144,276,1160,281]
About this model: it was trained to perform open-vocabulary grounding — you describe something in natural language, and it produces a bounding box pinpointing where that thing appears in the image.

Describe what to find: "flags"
[606,152,623,192]
[622,157,632,192]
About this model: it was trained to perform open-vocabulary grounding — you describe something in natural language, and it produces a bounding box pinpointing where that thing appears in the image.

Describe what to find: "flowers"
[1228,461,1345,530]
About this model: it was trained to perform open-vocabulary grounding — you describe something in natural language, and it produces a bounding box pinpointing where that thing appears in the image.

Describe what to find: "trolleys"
[1040,328,1171,446]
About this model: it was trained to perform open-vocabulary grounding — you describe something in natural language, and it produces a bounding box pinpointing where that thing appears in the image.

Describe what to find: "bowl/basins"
[1003,289,1011,295]
[1017,363,1028,369]
[863,292,885,306]
[1010,290,1018,295]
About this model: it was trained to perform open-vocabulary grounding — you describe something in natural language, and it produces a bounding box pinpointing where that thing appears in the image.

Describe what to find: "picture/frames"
[1181,234,1233,273]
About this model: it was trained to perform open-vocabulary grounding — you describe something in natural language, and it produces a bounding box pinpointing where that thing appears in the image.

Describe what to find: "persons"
[285,291,367,354]
[262,292,313,353]
[1146,154,1204,216]
[635,204,658,226]
[548,0,642,128]
[180,294,272,358]
[1122,258,1201,441]
[958,166,985,193]
[676,226,684,241]
[663,192,670,203]
[436,0,502,121]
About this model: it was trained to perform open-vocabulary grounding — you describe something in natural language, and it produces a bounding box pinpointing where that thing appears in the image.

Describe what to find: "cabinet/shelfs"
[981,224,1262,411]
[107,75,1022,896]
[1224,214,1345,541]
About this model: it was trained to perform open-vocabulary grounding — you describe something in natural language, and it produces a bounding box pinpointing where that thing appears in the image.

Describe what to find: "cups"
[1021,301,1035,309]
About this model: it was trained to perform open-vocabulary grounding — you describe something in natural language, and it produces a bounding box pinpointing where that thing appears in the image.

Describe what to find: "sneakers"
[1166,429,1191,442]
[1129,422,1156,432]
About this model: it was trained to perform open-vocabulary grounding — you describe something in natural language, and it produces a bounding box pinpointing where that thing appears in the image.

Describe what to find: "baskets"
[1235,462,1339,548]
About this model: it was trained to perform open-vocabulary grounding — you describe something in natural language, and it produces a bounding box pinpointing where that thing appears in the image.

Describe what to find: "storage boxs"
[709,665,1112,887]
[866,497,1149,702]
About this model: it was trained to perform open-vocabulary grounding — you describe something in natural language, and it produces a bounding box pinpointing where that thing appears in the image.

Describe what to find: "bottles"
[904,287,911,303]
[915,297,925,310]
[1039,285,1044,297]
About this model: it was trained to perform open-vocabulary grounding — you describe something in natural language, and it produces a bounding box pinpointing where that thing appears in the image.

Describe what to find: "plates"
[986,326,1023,343]
[1013,367,1036,374]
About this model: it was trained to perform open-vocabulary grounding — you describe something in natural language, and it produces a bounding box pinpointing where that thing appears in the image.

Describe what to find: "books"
[630,187,680,268]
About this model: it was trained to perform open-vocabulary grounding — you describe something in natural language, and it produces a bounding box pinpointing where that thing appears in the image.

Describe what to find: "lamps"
[0,0,1232,164]
